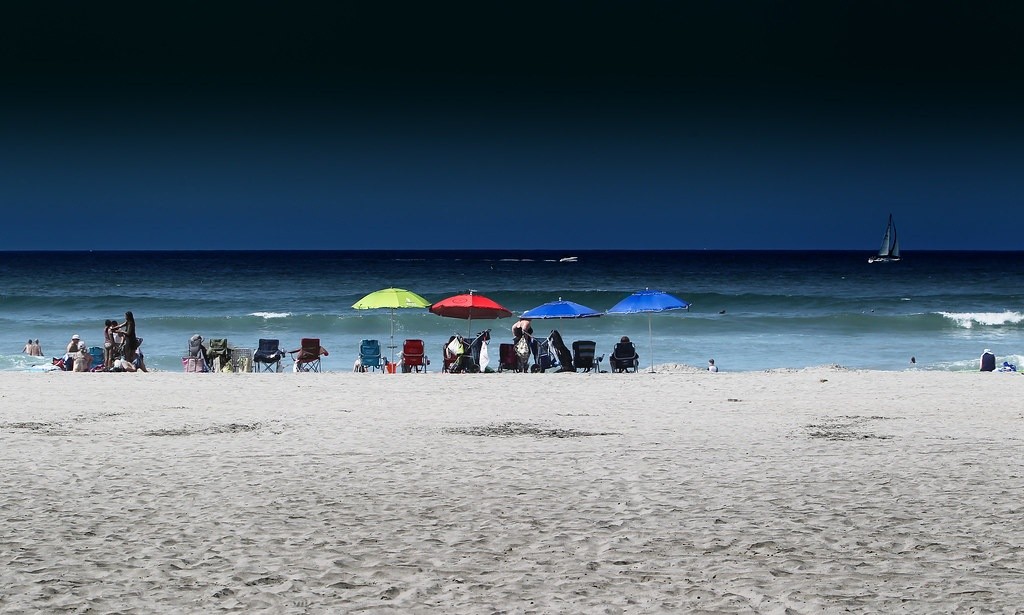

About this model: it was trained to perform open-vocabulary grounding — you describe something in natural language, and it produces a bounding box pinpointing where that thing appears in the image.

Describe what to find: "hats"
[71,334,80,339]
[191,333,204,343]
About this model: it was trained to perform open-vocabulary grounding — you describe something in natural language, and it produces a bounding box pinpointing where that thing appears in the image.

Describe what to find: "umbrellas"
[429,291,512,344]
[606,287,692,373]
[517,296,604,319]
[351,286,432,374]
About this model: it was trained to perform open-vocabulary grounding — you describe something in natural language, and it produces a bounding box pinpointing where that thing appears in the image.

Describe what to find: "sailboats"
[869,212,901,263]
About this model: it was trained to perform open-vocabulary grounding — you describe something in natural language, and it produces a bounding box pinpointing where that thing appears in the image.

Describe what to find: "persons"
[22,310,137,372]
[612,336,639,358]
[512,310,533,372]
[707,359,718,372]
[980,349,995,372]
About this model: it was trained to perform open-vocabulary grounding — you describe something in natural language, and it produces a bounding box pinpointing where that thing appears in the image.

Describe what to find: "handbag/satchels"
[104,343,112,350]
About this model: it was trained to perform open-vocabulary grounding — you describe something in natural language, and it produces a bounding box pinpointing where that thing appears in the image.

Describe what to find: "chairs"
[442,344,458,372]
[572,341,600,372]
[87,346,106,371]
[187,339,208,372]
[499,343,518,373]
[610,341,640,373]
[206,338,231,372]
[254,338,287,373]
[402,340,427,373]
[533,337,556,371]
[356,339,384,373]
[462,338,481,370]
[289,338,329,373]
[981,353,995,372]
[231,348,252,372]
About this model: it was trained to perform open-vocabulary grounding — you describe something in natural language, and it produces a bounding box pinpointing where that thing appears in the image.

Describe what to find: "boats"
[52,347,143,372]
[560,256,578,262]
